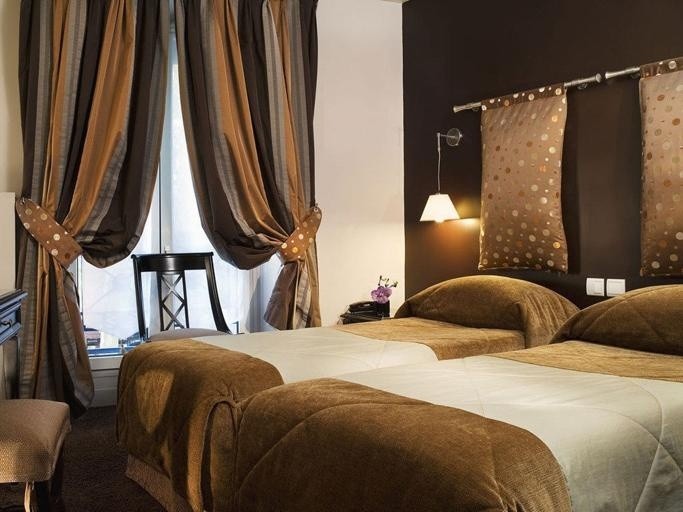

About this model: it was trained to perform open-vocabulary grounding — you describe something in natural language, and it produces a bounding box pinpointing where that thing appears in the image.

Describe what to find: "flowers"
[371,275,398,304]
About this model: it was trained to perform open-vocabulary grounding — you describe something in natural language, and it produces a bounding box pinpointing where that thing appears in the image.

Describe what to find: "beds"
[116,275,580,512]
[232,283,682,512]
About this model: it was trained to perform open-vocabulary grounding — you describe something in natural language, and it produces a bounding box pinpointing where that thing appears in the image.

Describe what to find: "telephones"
[348,301,377,313]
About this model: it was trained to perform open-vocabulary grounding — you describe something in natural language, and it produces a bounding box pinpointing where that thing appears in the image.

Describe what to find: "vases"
[373,300,390,317]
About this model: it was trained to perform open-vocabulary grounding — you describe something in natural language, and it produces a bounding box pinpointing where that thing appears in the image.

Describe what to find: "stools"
[0,399,71,512]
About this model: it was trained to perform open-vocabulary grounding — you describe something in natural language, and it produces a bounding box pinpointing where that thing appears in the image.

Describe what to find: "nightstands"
[340,312,393,324]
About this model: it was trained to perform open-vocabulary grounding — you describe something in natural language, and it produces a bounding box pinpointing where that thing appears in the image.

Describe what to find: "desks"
[0,288,28,398]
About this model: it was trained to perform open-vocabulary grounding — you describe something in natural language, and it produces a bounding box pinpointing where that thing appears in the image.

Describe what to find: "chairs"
[131,252,244,344]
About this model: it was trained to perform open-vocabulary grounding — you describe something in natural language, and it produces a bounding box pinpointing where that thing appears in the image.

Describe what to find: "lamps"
[419,128,463,223]
